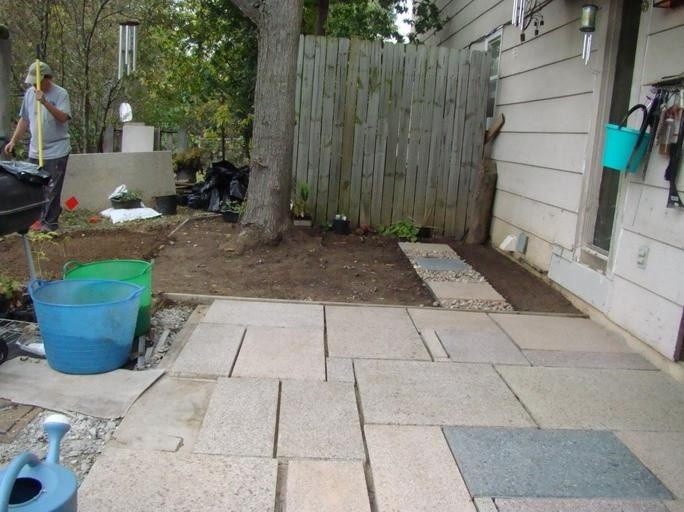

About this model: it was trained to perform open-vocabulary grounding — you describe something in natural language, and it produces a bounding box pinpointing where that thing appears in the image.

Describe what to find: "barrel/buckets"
[62,259,155,336]
[600,103,652,172]
[27,278,145,374]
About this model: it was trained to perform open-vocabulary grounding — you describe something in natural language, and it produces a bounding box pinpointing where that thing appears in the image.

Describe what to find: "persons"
[4,62,72,234]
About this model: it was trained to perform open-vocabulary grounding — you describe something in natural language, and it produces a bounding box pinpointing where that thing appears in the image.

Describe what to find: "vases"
[333,221,350,235]
[152,195,178,215]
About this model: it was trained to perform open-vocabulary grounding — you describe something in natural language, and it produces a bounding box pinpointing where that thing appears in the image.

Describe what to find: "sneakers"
[40,223,58,233]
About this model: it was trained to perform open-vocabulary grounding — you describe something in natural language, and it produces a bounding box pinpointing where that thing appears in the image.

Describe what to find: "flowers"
[334,209,351,221]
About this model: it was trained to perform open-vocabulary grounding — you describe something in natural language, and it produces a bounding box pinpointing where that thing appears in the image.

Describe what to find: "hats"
[24,62,52,84]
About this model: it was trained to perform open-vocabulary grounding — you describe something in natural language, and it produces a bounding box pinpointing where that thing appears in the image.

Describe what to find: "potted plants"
[218,198,240,223]
[110,186,143,210]
[291,182,312,226]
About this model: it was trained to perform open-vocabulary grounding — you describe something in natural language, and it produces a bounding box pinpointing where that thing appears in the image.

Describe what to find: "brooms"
[35,59,55,221]
